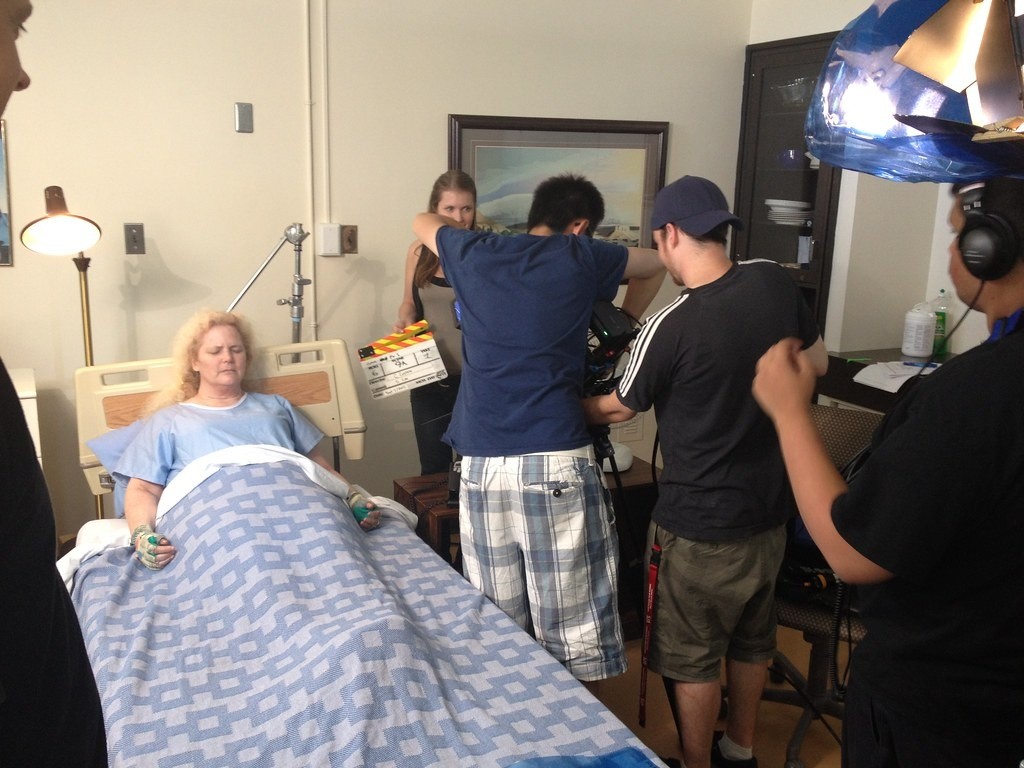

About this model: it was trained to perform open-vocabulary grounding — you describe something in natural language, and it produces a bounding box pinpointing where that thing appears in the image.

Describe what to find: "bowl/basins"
[777,149,809,168]
[767,76,817,110]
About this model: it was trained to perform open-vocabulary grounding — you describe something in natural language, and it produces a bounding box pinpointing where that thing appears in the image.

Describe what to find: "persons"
[412,176,666,696]
[584,175,828,768]
[116,310,409,642]
[0,1,109,768]
[752,138,1024,768]
[394,170,486,475]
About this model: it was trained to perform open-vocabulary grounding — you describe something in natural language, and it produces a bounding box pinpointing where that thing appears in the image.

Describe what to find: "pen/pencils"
[902,362,939,367]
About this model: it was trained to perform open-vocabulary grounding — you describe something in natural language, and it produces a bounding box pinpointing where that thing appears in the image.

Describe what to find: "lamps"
[20,188,110,367]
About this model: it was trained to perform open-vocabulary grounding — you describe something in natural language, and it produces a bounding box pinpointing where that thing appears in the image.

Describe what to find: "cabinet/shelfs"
[728,31,851,343]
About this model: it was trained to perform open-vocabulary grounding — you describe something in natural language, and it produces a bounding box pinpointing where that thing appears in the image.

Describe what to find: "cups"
[900,313,938,358]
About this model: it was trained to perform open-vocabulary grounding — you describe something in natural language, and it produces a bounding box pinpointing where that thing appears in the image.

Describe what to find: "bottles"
[930,289,953,356]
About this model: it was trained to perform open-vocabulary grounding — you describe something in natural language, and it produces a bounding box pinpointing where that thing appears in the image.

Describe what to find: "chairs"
[712,405,894,768]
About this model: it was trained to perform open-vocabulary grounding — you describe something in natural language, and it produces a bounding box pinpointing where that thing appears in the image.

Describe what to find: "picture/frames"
[0,119,12,266]
[447,114,671,285]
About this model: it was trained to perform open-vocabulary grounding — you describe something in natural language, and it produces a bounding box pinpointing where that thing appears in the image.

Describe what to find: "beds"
[74,340,671,768]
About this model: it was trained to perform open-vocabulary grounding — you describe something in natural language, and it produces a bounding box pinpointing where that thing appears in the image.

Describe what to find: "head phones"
[959,176,1019,282]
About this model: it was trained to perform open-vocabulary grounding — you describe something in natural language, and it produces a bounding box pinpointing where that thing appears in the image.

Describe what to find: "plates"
[764,198,814,225]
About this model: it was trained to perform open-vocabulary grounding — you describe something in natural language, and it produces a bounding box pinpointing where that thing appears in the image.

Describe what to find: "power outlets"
[125,224,145,254]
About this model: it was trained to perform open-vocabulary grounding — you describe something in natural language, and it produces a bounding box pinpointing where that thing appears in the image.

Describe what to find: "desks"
[815,347,960,416]
[394,455,662,639]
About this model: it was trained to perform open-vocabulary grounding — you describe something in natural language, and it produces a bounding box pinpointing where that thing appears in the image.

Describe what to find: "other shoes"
[658,757,682,768]
[711,729,758,768]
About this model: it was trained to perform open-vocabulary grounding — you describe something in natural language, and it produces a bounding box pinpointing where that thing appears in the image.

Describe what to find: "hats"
[652,174,744,237]
